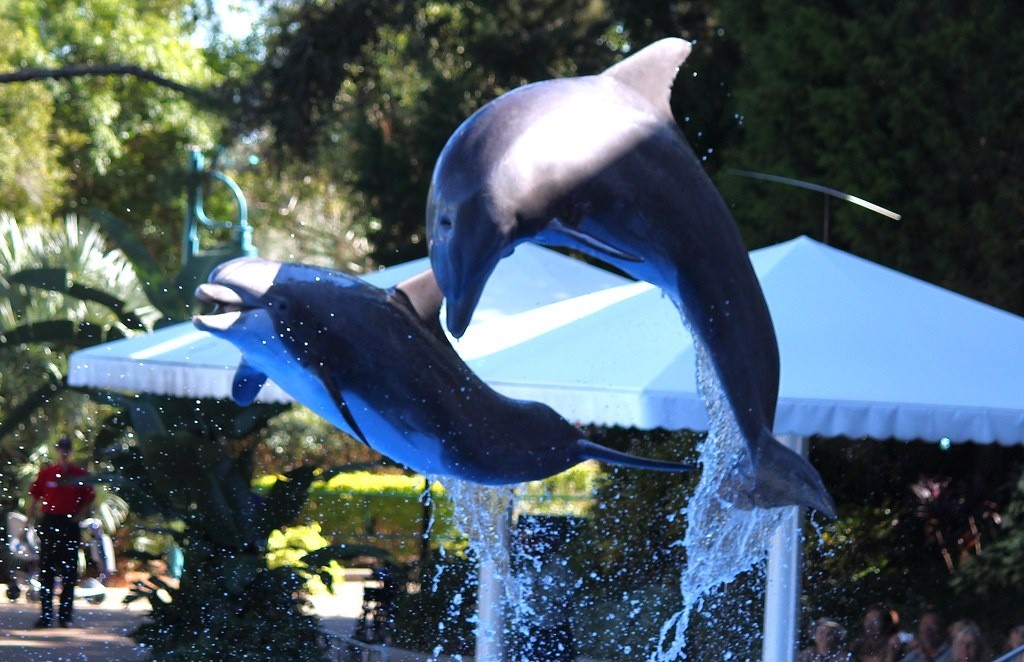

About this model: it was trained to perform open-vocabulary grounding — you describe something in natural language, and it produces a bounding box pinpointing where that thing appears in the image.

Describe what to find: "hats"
[55,438,74,453]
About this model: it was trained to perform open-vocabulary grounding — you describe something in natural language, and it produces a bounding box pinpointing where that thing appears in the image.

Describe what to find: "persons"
[795,603,1022,662]
[24,439,96,625]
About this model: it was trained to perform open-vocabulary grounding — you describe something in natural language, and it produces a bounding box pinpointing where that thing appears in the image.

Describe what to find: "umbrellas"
[67,237,637,662]
[442,232,1023,662]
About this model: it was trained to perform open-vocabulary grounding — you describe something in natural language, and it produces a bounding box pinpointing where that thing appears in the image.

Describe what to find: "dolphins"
[193,254,704,486]
[425,37,840,521]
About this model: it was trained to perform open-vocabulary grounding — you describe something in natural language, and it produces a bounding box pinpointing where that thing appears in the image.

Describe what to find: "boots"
[35,599,53,627]
[59,601,74,627]
